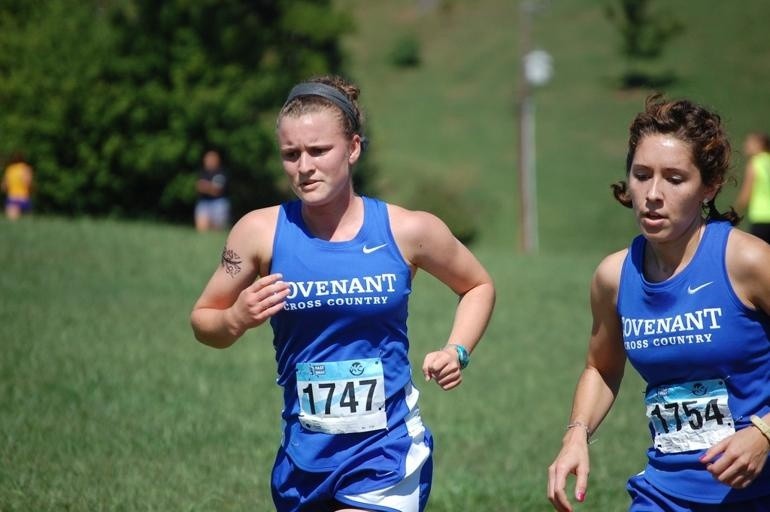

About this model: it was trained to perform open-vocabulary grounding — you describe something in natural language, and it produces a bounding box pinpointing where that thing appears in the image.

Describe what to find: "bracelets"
[750,414,770,442]
[565,421,598,446]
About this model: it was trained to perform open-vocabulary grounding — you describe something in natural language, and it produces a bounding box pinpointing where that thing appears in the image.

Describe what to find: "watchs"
[443,343,468,368]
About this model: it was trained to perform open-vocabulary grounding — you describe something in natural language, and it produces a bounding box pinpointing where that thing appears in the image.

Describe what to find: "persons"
[190,74,495,511]
[0,155,33,219]
[195,150,230,231]
[735,133,770,242]
[546,91,770,512]
